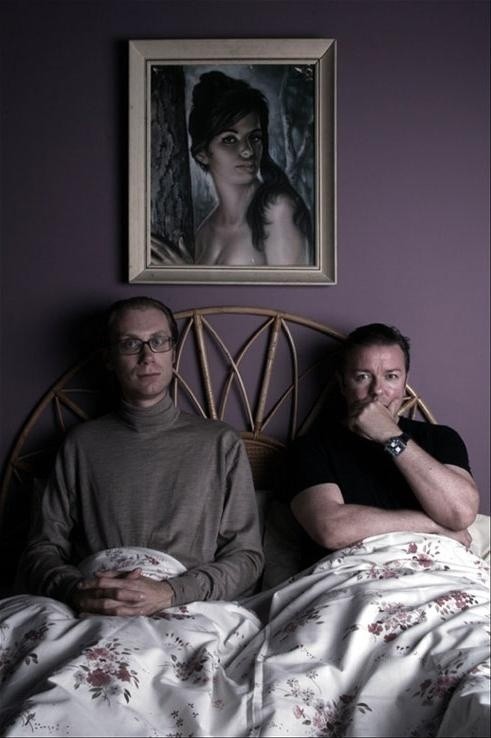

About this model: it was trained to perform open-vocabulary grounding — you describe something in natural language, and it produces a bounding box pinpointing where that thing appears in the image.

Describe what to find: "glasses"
[113,334,176,356]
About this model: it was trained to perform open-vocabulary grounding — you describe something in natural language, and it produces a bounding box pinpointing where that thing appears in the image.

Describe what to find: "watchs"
[381,433,410,457]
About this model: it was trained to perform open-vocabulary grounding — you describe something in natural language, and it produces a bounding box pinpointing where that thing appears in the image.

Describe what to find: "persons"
[20,295,265,617]
[149,70,316,266]
[272,322,480,549]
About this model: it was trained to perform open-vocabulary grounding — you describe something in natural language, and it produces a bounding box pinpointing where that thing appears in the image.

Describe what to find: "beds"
[0,306,491,738]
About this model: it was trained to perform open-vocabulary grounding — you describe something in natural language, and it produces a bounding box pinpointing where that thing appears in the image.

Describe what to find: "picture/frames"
[129,36,336,285]
